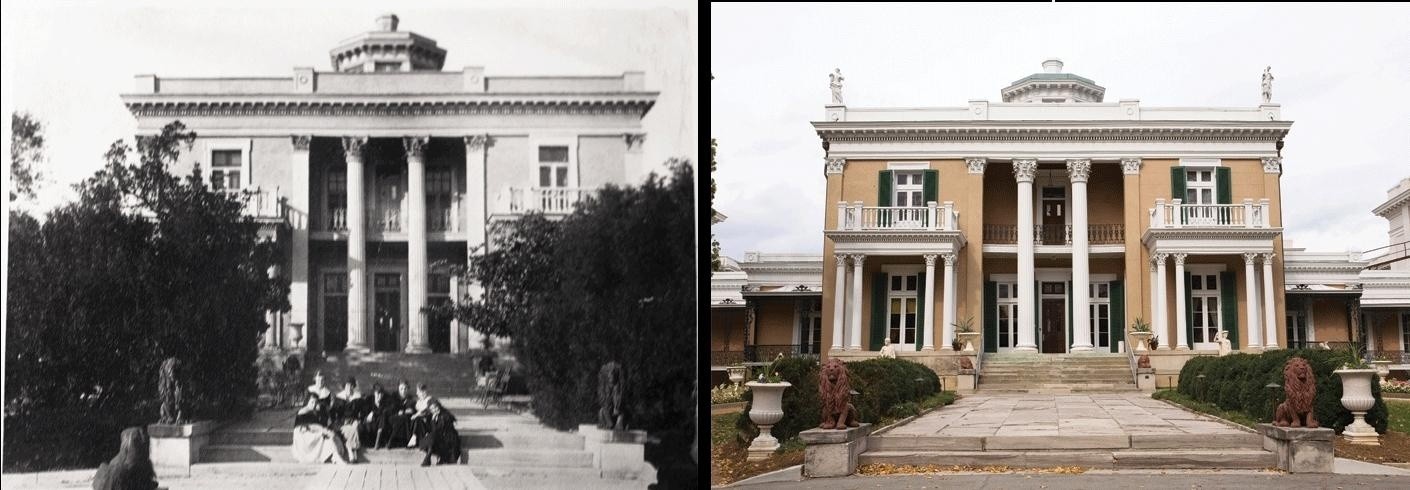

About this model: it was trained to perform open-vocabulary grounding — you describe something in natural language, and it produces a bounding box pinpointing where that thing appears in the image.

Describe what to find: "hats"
[342,375,358,389]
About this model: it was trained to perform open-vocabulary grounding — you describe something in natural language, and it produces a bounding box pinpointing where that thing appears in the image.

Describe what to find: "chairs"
[475,359,512,411]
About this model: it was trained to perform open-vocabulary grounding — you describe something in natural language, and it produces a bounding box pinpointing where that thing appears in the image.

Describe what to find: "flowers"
[1324,339,1373,369]
[744,347,784,383]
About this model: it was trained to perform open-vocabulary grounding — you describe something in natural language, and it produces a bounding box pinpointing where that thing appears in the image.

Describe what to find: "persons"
[1261,64,1274,103]
[879,337,896,359]
[828,65,844,105]
[1213,329,1233,357]
[1318,340,1332,351]
[286,362,461,472]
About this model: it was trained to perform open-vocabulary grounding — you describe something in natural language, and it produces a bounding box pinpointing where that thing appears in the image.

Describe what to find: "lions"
[1271,357,1320,429]
[598,362,634,431]
[817,358,860,430]
[156,356,195,425]
[90,428,158,490]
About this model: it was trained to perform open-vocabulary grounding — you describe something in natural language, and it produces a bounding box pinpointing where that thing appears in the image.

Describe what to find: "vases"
[1333,370,1381,447]
[745,380,792,462]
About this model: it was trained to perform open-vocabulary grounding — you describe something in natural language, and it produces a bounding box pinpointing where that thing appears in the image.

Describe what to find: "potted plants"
[952,314,981,351]
[1129,313,1153,351]
